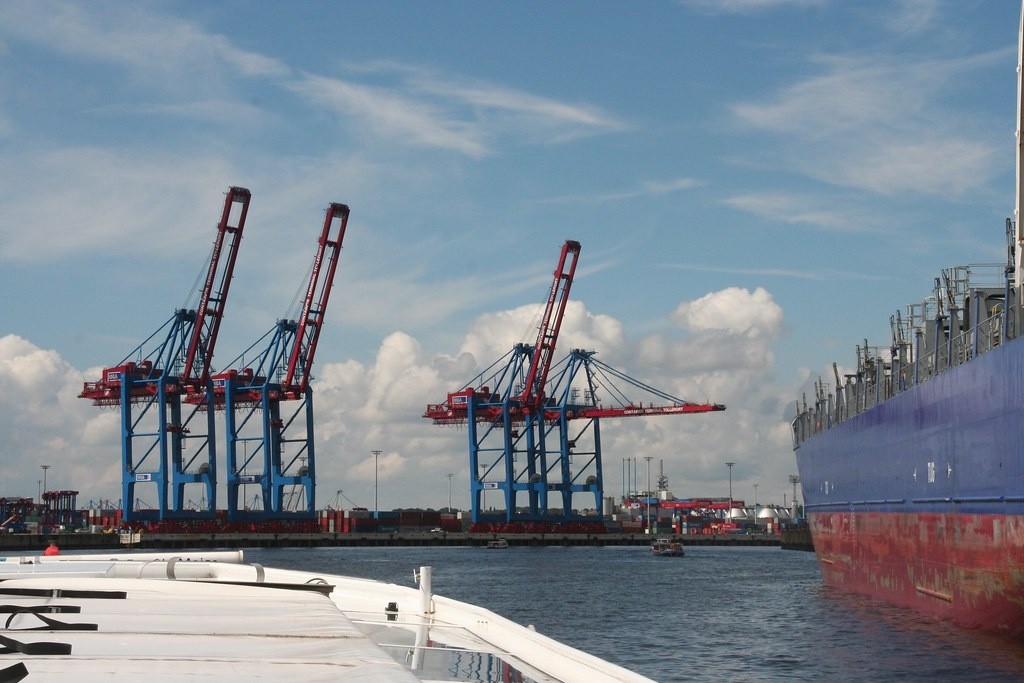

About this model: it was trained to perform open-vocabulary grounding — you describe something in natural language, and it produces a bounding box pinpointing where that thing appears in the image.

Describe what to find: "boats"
[650,535,685,557]
[0,545,659,683]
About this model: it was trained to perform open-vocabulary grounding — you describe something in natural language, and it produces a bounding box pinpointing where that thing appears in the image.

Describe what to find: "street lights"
[446,473,454,513]
[297,456,309,511]
[725,462,735,523]
[644,456,654,491]
[480,464,490,512]
[40,465,50,493]
[371,450,383,512]
[240,439,250,510]
[36,480,43,505]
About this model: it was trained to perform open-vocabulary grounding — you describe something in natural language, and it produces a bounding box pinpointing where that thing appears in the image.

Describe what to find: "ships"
[791,216,1023,641]
